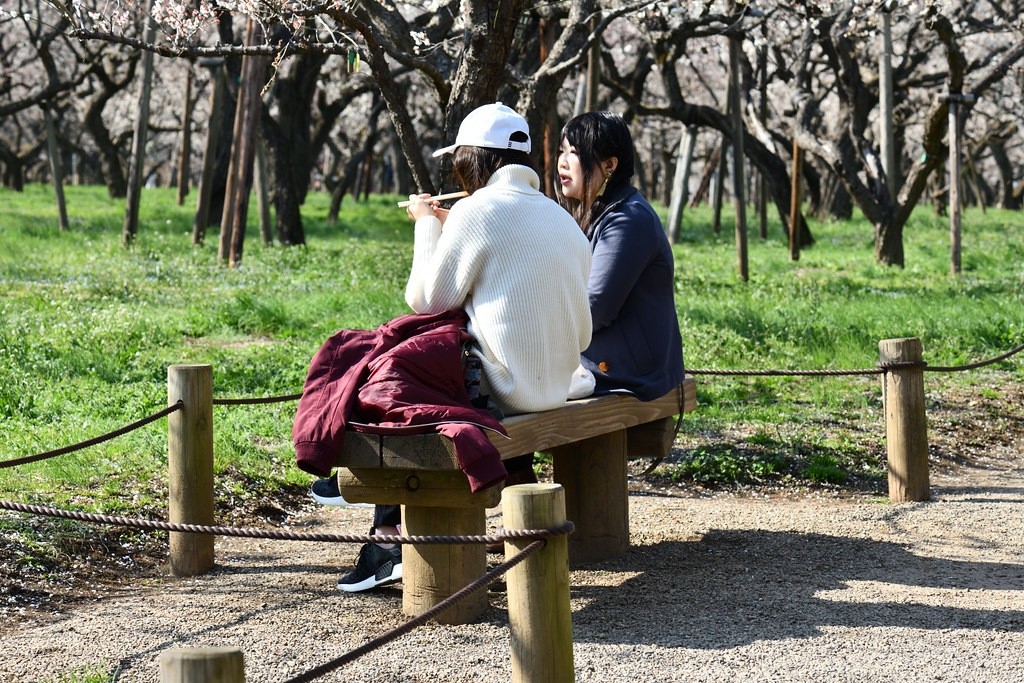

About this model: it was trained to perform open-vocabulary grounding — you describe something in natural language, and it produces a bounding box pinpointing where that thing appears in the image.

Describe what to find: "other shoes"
[485,540,505,553]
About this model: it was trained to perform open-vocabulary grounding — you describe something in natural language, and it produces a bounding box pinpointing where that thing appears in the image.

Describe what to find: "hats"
[432,102,532,158]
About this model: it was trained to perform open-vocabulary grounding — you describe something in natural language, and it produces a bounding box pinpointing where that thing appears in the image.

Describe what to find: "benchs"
[333,372,697,627]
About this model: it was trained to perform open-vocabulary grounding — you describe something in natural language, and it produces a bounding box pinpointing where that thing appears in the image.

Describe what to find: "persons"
[312,102,592,593]
[503,111,685,484]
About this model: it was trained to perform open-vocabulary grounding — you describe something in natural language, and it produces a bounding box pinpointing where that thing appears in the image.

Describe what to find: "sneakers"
[336,542,403,592]
[310,470,376,509]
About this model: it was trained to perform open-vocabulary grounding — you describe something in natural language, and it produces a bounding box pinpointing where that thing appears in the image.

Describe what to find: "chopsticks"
[397,191,469,209]
